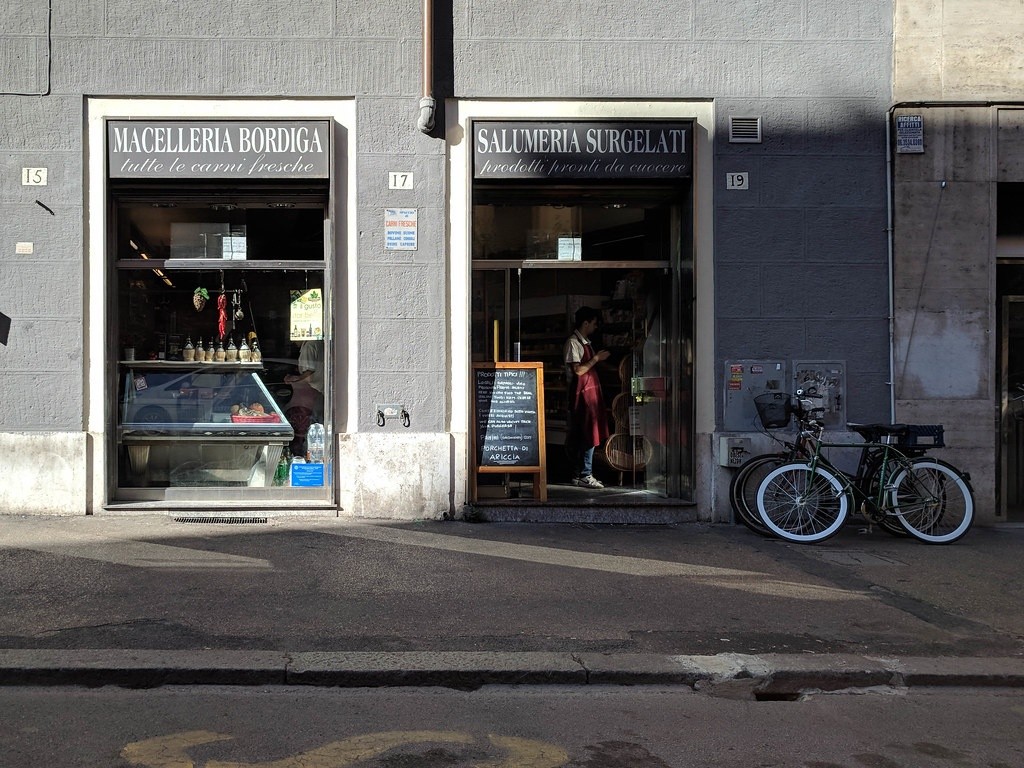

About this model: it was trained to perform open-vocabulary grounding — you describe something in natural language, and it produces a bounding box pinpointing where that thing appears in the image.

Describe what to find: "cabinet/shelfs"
[511,293,667,429]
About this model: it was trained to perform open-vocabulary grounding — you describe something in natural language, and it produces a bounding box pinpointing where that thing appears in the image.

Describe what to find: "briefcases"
[898,424,945,448]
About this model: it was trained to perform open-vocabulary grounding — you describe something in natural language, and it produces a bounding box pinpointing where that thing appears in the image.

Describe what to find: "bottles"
[307,423,325,449]
[182,333,261,362]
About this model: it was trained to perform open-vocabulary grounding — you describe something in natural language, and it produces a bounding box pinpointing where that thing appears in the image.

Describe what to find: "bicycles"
[729,387,976,546]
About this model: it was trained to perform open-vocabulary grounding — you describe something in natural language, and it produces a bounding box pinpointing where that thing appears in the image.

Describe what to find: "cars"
[126,360,322,451]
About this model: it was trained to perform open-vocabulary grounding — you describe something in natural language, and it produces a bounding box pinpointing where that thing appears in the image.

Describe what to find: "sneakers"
[578,474,604,489]
[572,475,603,486]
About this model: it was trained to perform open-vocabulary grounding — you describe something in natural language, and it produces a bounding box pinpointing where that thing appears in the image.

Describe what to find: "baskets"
[612,392,649,430]
[754,393,791,429]
[618,353,633,385]
[603,432,653,472]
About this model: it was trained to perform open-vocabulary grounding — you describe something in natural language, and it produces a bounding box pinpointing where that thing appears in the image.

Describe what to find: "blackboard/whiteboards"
[470,362,546,476]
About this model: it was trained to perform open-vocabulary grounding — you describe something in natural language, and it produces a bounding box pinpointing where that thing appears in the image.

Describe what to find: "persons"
[566,306,610,490]
[283,330,324,461]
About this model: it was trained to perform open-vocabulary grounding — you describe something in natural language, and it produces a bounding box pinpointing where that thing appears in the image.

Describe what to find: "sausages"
[218,296,227,339]
